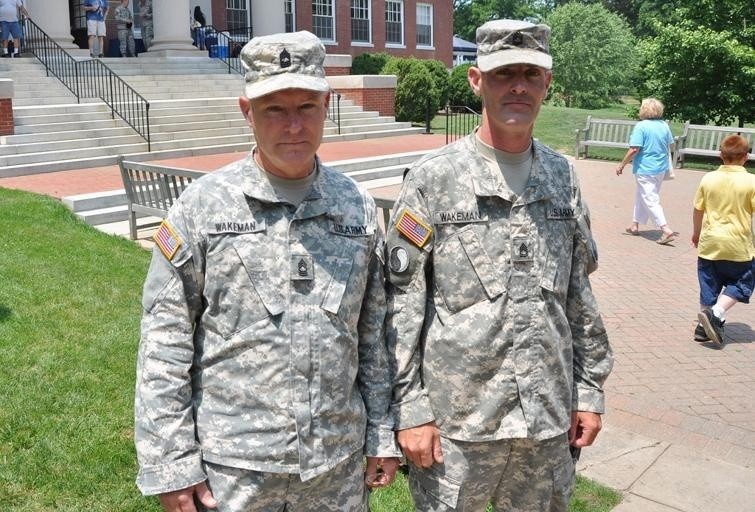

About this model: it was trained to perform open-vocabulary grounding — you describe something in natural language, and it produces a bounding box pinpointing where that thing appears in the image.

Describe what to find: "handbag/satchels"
[663,149,675,180]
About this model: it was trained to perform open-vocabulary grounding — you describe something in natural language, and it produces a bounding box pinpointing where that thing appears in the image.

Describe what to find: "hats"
[240,31,329,98]
[475,20,552,72]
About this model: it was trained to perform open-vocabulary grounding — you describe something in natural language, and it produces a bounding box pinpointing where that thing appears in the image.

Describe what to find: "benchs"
[371,196,400,232]
[571,113,674,161]
[671,118,755,172]
[114,154,212,246]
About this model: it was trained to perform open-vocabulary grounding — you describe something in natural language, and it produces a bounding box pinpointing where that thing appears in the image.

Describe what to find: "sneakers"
[695,308,724,345]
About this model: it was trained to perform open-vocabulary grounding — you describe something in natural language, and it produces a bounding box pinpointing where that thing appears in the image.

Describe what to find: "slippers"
[626,228,639,235]
[656,231,675,243]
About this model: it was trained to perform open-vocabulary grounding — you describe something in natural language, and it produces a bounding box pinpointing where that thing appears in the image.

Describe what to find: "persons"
[616,98,676,244]
[84,0,110,58]
[115,0,137,57]
[194,6,205,50]
[381,21,614,511]
[0,0,32,58]
[134,33,399,512]
[137,0,152,50]
[692,136,755,348]
